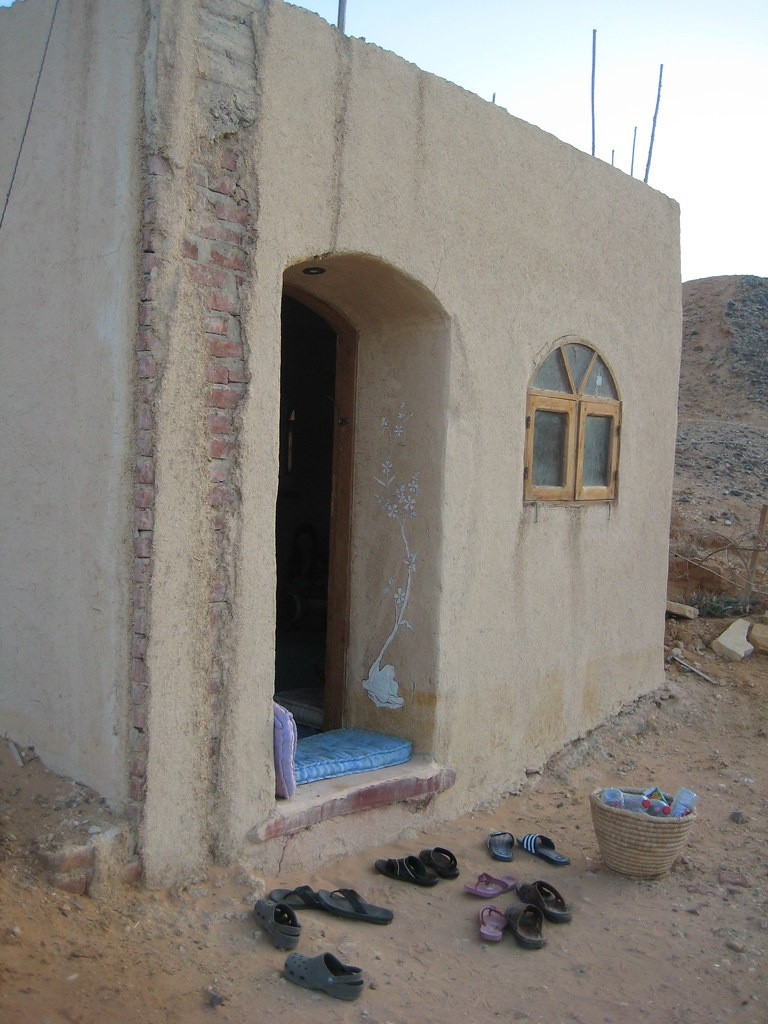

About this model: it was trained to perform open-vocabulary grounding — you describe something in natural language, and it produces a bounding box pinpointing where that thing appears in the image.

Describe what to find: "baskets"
[589,787,697,881]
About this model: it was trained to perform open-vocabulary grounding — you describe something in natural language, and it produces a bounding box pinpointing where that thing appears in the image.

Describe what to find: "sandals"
[283,951,364,1001]
[253,898,302,951]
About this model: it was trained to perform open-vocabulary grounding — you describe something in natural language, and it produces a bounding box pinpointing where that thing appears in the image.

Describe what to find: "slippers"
[375,855,439,887]
[504,902,544,949]
[464,872,519,898]
[270,885,329,910]
[516,880,572,923]
[418,846,460,880]
[486,831,515,862]
[516,833,570,865]
[317,888,394,925]
[478,904,507,942]
